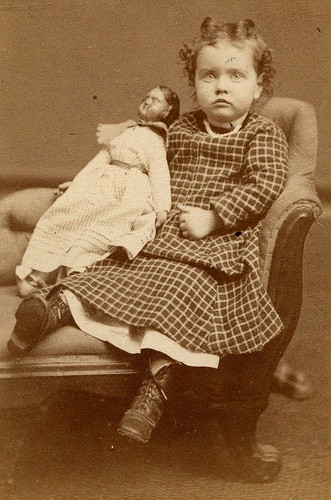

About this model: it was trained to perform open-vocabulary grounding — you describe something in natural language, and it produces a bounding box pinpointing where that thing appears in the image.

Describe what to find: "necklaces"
[202,119,242,138]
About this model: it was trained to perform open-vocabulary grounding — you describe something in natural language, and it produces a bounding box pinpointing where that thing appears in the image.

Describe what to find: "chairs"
[0,96,331,482]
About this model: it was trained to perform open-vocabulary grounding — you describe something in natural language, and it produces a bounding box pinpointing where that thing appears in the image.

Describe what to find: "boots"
[6,285,72,356]
[116,356,175,444]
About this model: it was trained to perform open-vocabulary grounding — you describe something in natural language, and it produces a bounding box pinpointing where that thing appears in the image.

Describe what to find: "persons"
[14,84,182,297]
[7,14,289,444]
